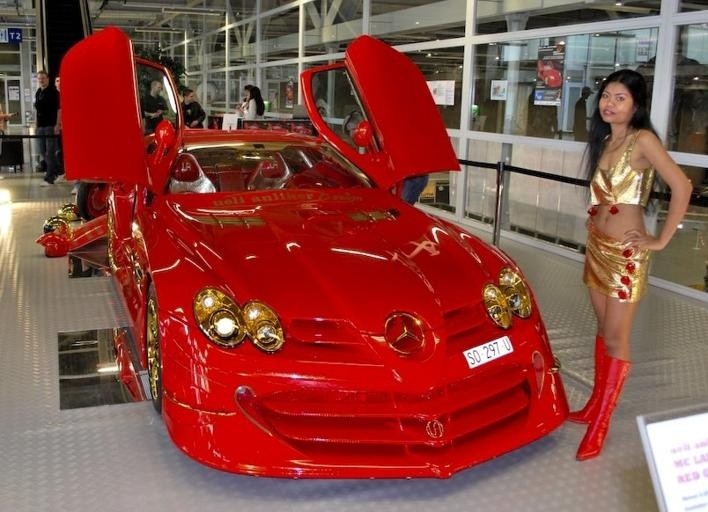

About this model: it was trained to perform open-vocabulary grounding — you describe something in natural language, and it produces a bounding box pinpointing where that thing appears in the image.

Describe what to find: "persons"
[483,97,505,136]
[566,68,694,462]
[1,72,14,180]
[241,84,252,108]
[314,86,332,123]
[573,85,595,144]
[142,79,169,133]
[244,87,264,120]
[54,74,66,177]
[180,89,206,128]
[343,112,428,207]
[34,69,62,187]
[527,87,560,139]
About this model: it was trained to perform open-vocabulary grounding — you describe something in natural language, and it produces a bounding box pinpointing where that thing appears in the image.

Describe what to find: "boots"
[566,336,630,460]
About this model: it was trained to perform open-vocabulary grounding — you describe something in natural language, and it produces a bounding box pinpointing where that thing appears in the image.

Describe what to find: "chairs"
[168,150,296,193]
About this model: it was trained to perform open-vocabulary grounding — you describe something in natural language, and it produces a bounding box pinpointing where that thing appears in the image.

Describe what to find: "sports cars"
[53,25,568,478]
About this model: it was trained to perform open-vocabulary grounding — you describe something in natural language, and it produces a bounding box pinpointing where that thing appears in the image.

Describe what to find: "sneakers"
[40,181,53,187]
[54,175,64,184]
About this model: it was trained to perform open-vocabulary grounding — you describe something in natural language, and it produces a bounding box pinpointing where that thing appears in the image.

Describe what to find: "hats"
[583,87,594,94]
